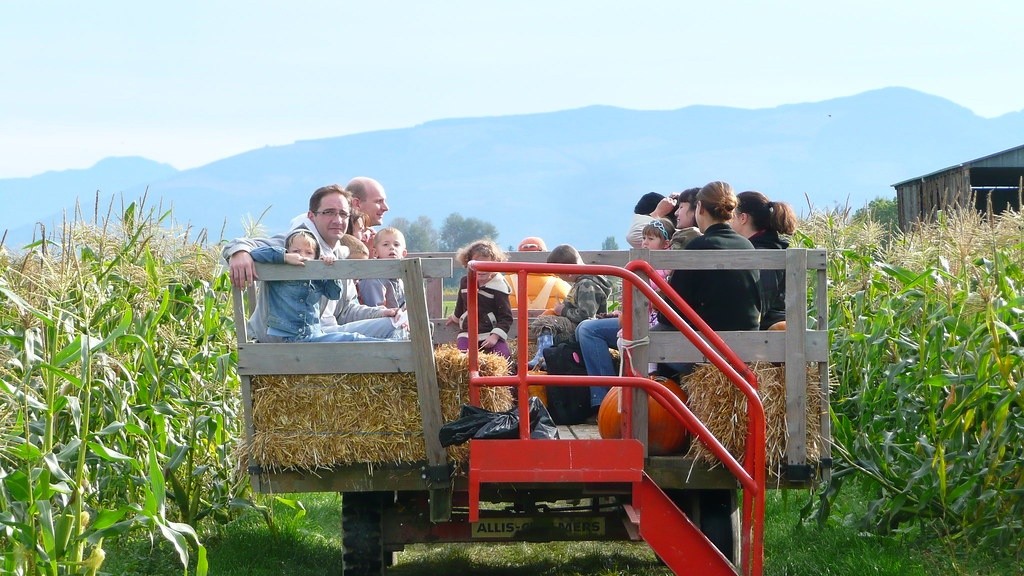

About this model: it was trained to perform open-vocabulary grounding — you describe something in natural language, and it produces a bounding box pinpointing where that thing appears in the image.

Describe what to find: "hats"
[518,236,547,251]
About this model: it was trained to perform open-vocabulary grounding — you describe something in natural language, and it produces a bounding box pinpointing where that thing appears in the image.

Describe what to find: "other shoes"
[586,412,598,425]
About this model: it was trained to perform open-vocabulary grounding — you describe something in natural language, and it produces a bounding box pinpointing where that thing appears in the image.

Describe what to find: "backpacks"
[543,339,593,425]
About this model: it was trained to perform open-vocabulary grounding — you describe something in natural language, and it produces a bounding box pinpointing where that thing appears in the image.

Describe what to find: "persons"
[502,237,574,310]
[616,219,676,375]
[250,228,391,342]
[344,177,389,284]
[728,192,795,331]
[575,187,704,426]
[220,184,408,342]
[445,241,516,408]
[527,244,613,370]
[340,233,369,308]
[649,182,762,376]
[347,207,378,259]
[358,227,407,312]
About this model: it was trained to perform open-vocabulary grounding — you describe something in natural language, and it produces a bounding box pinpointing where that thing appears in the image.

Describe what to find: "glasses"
[314,211,350,219]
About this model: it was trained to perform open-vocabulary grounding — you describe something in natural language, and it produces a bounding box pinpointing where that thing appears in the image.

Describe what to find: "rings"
[670,198,671,201]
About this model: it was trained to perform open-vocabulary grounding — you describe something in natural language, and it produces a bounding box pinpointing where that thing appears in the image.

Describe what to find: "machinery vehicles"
[232,247,829,576]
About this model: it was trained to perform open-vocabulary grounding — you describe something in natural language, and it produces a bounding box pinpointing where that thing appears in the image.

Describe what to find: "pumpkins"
[597,377,689,455]
[767,321,788,366]
[526,358,548,408]
